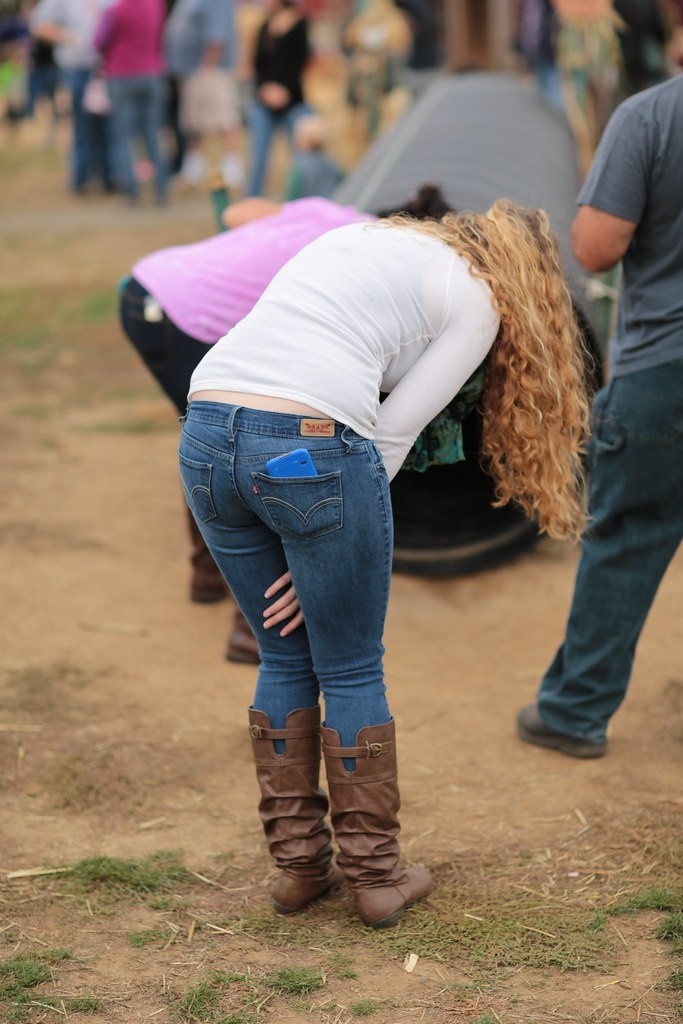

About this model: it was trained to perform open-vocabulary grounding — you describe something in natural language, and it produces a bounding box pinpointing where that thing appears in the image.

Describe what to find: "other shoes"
[516,704,607,760]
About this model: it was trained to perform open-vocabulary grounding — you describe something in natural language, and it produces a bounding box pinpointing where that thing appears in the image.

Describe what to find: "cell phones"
[266,449,318,479]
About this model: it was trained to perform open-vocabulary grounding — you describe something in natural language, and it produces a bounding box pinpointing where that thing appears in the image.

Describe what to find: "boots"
[227,609,261,664]
[187,505,229,603]
[246,704,347,915]
[319,716,432,929]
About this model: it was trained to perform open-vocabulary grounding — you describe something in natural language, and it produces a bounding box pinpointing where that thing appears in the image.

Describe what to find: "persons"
[181,200,595,929]
[121,185,453,664]
[0,0,683,212]
[519,72,683,759]
